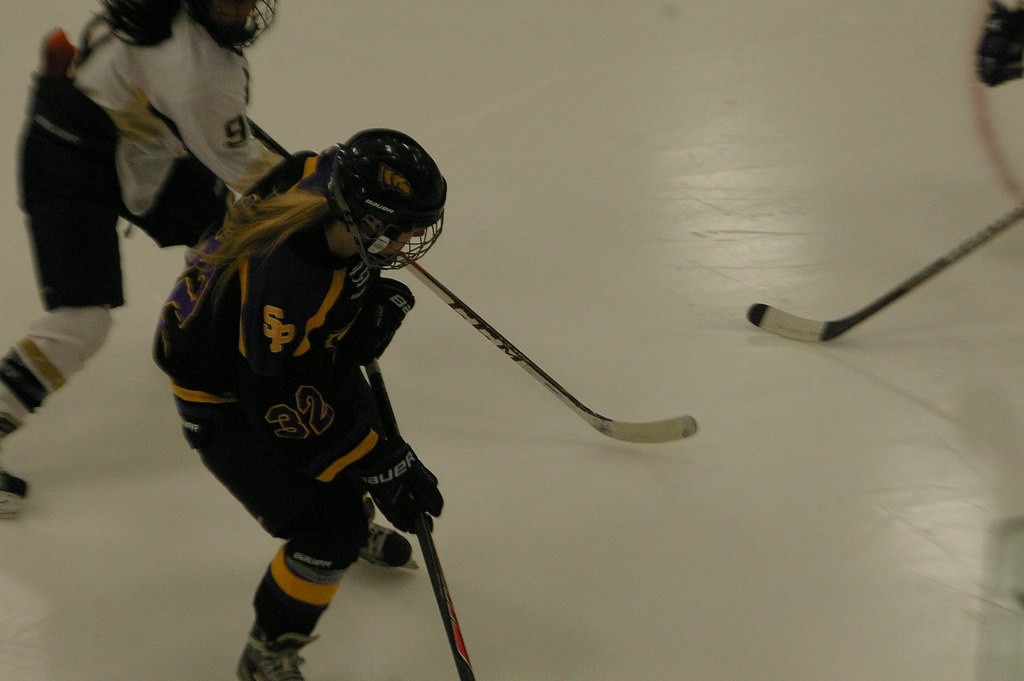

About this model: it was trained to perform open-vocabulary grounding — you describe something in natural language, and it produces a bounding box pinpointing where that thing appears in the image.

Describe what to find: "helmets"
[187,0,277,50]
[330,128,447,270]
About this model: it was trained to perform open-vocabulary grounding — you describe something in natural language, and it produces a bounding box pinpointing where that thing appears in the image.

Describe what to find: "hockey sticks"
[245,116,700,443]
[367,358,477,681]
[746,197,1023,342]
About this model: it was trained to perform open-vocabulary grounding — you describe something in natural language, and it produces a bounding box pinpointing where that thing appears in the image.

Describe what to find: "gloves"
[338,278,415,368]
[360,443,444,534]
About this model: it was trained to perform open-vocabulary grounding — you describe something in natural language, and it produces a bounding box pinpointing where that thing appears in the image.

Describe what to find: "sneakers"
[236,623,320,681]
[0,412,27,517]
[358,522,420,570]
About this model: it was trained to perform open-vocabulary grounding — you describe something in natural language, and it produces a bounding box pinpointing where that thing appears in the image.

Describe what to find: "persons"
[151,128,448,681]
[0,0,285,519]
